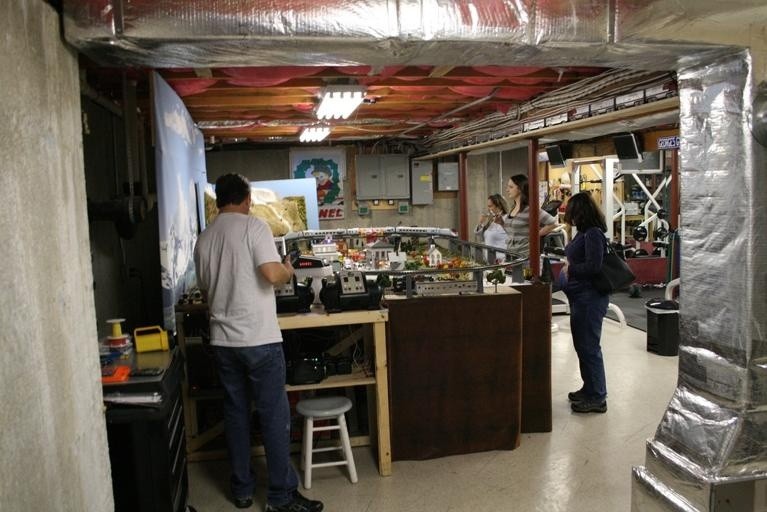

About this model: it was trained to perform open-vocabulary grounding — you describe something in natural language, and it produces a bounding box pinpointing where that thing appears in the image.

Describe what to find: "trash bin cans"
[644,297,679,356]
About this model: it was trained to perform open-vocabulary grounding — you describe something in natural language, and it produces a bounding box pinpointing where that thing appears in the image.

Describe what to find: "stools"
[296,396,358,489]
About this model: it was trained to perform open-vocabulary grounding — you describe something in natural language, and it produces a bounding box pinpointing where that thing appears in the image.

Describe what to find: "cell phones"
[130,367,163,376]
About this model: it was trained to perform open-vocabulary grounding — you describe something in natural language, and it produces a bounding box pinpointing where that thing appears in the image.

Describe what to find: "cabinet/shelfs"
[100,346,189,512]
[614,215,653,241]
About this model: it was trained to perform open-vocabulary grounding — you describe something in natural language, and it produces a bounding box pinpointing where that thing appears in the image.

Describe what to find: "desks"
[175,304,392,477]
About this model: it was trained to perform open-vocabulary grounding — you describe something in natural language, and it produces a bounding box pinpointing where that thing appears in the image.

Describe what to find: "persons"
[311,167,333,200]
[564,191,609,413]
[474,194,510,272]
[194,173,323,512]
[494,174,558,281]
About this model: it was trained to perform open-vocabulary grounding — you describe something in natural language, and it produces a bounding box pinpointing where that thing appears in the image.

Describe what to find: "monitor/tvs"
[612,133,642,164]
[545,144,566,169]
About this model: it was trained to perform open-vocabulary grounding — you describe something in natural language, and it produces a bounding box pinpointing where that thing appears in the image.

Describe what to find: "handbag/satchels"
[591,253,636,295]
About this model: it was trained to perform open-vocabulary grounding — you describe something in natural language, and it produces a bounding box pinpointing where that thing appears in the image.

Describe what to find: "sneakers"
[235,498,252,508]
[571,395,607,412]
[569,389,584,401]
[265,490,323,512]
